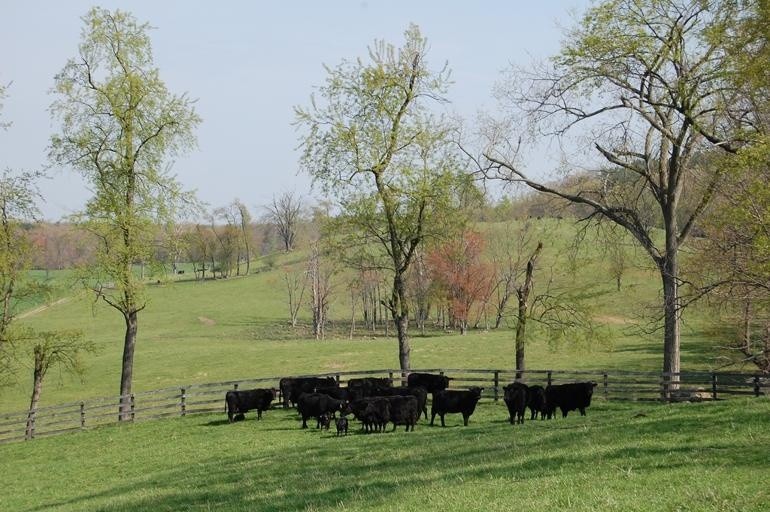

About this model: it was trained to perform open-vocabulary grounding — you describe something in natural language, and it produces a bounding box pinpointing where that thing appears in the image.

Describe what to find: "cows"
[408,373,453,395]
[503,382,598,425]
[430,387,484,428]
[225,388,280,424]
[279,376,427,436]
